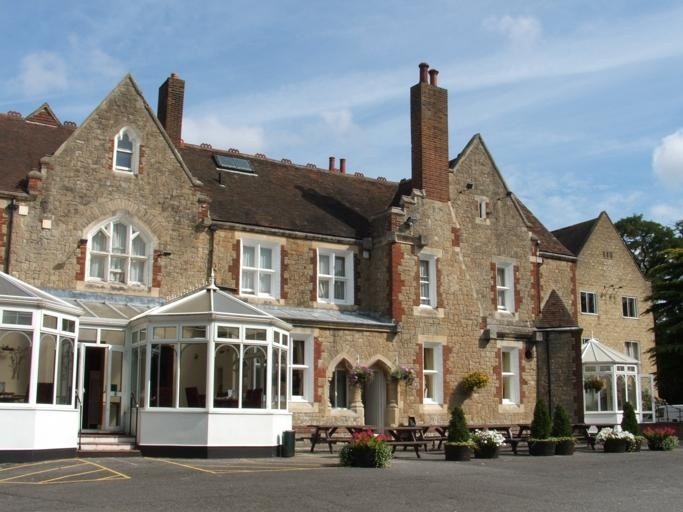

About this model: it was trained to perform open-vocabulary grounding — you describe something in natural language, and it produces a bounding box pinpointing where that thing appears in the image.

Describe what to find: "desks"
[437,422,614,455]
[307,424,429,458]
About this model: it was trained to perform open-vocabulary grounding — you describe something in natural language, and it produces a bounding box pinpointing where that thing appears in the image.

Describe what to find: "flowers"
[387,365,417,385]
[347,366,375,388]
[596,423,636,443]
[462,371,490,393]
[640,425,680,450]
[584,375,607,394]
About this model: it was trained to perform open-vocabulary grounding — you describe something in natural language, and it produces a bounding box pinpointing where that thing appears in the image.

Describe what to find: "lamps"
[77,238,87,248]
[159,251,171,257]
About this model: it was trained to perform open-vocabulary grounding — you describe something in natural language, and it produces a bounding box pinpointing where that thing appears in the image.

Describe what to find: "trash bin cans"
[282,431,295,457]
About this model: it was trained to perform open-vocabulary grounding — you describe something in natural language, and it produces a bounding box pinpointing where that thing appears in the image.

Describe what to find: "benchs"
[304,435,426,459]
[438,433,597,454]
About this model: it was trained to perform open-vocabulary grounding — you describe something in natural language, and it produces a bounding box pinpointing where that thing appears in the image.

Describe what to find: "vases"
[349,446,377,466]
[604,437,627,453]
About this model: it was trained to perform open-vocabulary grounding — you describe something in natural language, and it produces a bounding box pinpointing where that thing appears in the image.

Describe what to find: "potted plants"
[526,399,576,455]
[445,399,469,461]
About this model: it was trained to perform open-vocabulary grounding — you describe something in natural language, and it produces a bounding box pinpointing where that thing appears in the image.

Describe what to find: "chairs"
[185,386,263,408]
